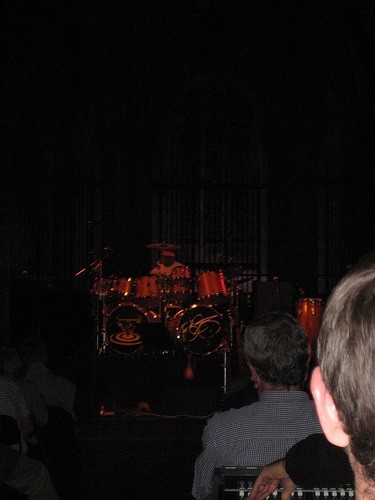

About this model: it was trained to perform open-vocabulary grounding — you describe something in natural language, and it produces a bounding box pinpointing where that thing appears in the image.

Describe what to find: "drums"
[106,278,133,302]
[196,270,228,306]
[93,278,110,298]
[105,302,149,355]
[168,305,225,356]
[136,274,163,306]
[296,298,324,340]
[167,278,190,303]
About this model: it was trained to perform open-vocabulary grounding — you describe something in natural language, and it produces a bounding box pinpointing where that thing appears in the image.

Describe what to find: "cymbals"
[86,250,120,256]
[146,243,182,250]
[229,278,253,287]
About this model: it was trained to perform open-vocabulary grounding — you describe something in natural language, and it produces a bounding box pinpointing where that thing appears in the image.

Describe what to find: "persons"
[191,312,324,500]
[149,254,187,277]
[252,261,375,500]
[0,344,77,500]
